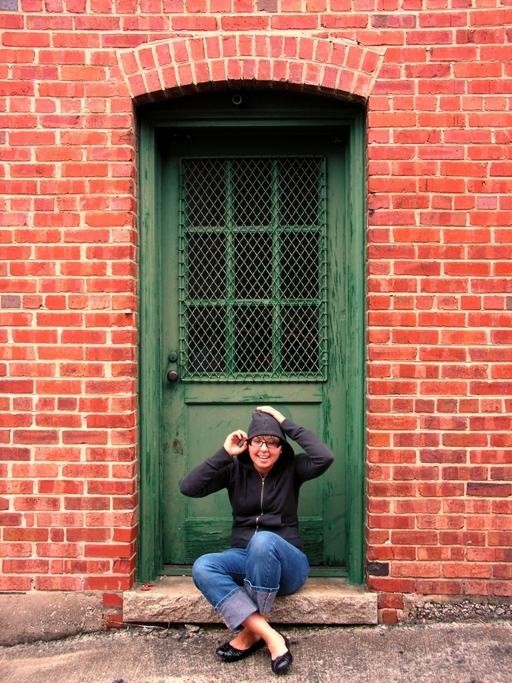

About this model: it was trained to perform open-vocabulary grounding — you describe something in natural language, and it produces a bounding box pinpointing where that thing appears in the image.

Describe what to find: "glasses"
[247,435,283,449]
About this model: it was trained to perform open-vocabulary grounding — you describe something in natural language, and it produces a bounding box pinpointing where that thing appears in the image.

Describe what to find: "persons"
[180,406,334,674]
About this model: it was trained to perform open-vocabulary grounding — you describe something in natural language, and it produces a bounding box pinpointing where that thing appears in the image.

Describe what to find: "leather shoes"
[215,636,267,662]
[270,631,293,675]
